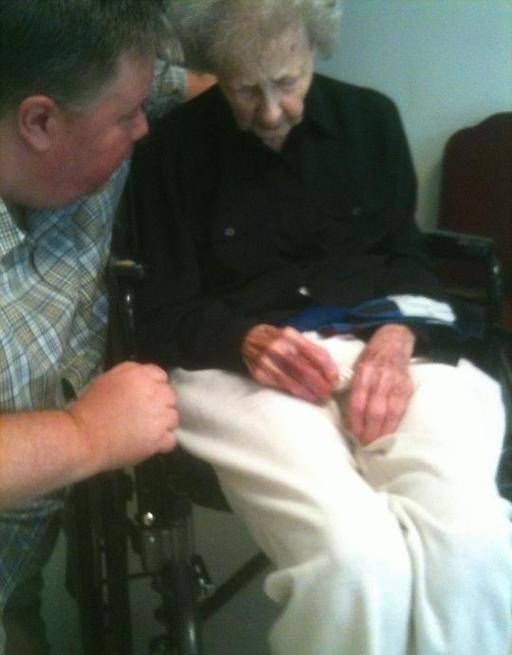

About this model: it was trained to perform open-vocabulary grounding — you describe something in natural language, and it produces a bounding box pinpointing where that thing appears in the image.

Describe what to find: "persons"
[103,0,512,655]
[0,0,224,654]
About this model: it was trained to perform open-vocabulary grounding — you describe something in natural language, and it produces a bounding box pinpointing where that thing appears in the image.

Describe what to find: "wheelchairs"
[65,221,512,655]
